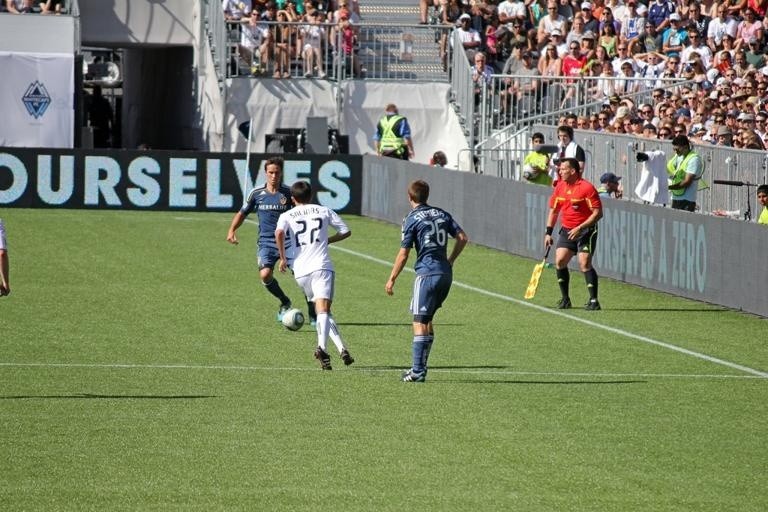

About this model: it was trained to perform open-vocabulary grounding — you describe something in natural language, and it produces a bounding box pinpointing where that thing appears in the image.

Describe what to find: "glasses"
[690,35,700,39]
[251,3,349,17]
[618,48,627,52]
[689,9,700,13]
[673,146,686,152]
[570,46,580,50]
[547,7,558,10]
[648,57,658,61]
[744,13,754,17]
[669,61,679,66]
[722,39,732,43]
[513,26,522,29]
[646,25,656,30]
[717,10,728,14]
[602,11,611,16]
[559,73,768,145]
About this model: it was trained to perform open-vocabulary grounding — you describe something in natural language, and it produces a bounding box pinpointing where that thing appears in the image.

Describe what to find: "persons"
[372,104,414,160]
[1,218,11,297]
[84,86,113,149]
[5,1,32,15]
[38,0,64,16]
[385,179,467,381]
[227,156,317,325]
[544,157,603,310]
[222,0,362,77]
[275,181,354,371]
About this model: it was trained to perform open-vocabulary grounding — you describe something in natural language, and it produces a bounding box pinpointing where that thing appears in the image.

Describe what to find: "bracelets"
[546,227,553,235]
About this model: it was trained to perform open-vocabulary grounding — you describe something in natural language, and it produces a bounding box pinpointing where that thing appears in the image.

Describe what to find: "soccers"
[282,309,303,331]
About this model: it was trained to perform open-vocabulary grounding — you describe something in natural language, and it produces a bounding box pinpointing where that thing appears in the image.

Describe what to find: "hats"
[459,12,471,20]
[748,37,760,45]
[669,13,682,23]
[706,68,720,82]
[551,30,563,37]
[581,2,593,11]
[522,51,533,58]
[600,172,623,184]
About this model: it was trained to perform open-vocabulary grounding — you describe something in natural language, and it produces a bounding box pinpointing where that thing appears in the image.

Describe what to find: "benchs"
[201,0,369,77]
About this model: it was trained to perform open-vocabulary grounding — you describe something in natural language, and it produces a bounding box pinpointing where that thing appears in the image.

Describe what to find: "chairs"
[431,0,686,141]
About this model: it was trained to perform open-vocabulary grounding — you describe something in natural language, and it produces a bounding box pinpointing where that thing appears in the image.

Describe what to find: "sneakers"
[557,296,573,309]
[399,365,428,384]
[339,349,356,367]
[309,311,318,325]
[584,296,602,309]
[313,345,333,373]
[277,299,292,321]
